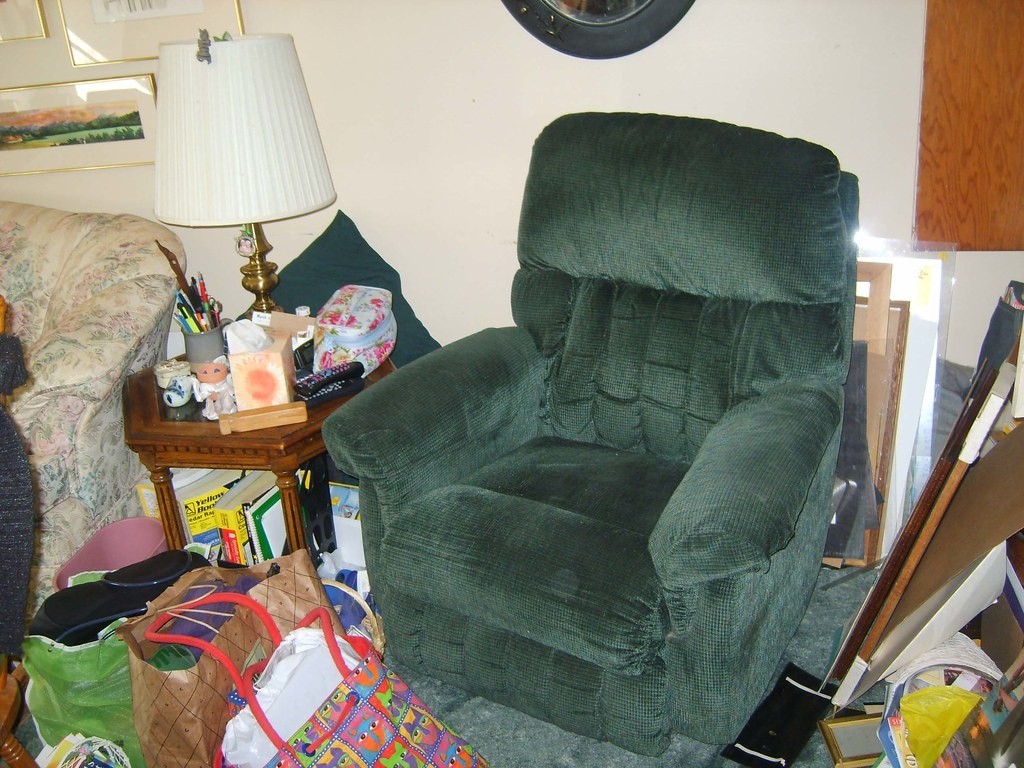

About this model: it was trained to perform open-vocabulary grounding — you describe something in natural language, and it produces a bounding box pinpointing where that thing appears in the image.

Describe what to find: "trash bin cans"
[53,516,169,593]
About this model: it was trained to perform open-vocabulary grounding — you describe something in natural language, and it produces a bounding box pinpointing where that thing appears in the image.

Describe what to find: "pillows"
[235,210,442,486]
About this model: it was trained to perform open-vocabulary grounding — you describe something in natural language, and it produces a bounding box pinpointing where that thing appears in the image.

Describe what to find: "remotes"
[294,377,365,408]
[294,361,364,398]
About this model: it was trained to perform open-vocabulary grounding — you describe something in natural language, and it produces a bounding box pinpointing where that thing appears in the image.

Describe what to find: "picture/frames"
[0,0,50,44]
[58,0,244,69]
[0,73,157,177]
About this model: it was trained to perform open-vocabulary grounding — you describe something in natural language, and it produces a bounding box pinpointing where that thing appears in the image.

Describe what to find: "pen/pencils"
[173,272,221,334]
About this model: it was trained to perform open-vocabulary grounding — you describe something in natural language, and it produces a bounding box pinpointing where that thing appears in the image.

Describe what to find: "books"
[136,464,364,567]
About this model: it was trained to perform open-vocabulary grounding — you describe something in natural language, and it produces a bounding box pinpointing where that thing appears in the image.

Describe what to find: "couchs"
[0,200,187,666]
[322,112,860,757]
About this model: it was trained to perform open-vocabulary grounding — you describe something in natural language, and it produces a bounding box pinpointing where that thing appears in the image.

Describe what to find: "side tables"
[121,357,396,555]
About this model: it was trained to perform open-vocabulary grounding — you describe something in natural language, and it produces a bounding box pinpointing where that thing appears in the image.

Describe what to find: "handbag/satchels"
[310,285,399,379]
[146,592,489,768]
[121,553,343,768]
[22,543,208,768]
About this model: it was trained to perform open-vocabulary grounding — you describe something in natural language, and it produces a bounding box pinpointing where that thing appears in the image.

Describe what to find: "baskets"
[321,579,386,654]
[879,633,1010,723]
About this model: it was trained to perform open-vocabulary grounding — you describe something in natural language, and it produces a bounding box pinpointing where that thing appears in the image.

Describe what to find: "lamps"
[155,32,337,317]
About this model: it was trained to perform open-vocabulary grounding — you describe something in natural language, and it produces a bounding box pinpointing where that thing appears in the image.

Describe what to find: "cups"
[198,362,227,402]
[181,318,234,363]
